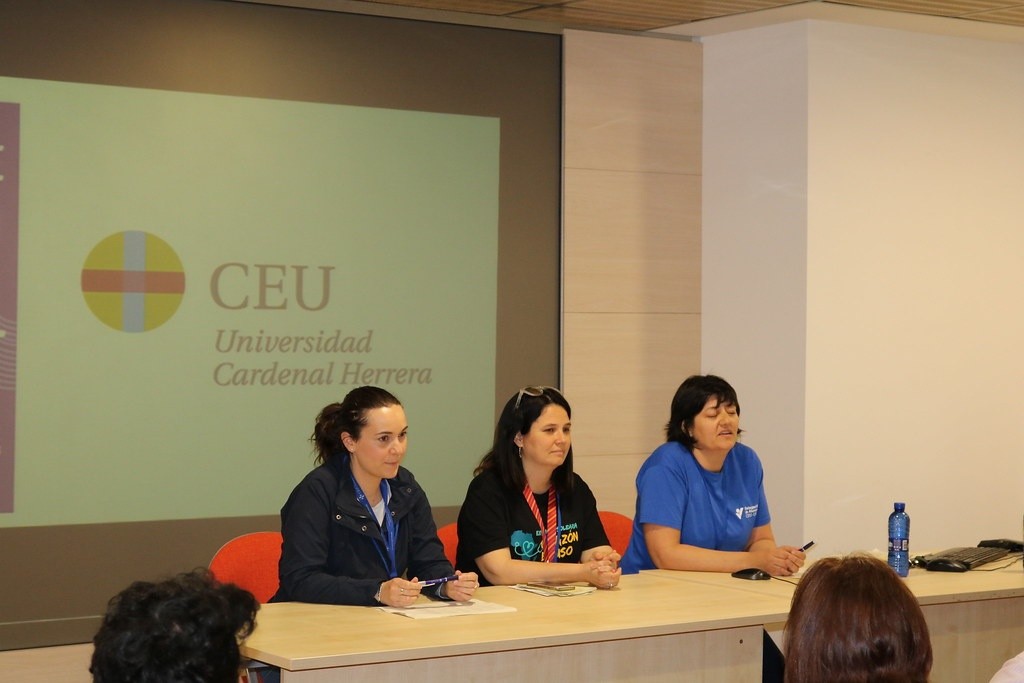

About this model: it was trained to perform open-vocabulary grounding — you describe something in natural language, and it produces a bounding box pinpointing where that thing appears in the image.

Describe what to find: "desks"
[231,551,1024,683]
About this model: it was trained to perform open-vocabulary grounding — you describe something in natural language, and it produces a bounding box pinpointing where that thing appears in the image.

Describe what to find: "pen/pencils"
[417,575,458,587]
[797,541,813,551]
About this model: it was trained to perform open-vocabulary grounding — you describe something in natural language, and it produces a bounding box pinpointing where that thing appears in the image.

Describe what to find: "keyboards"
[921,547,1011,570]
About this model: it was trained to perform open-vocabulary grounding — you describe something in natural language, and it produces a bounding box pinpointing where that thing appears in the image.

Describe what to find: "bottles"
[887,502,910,578]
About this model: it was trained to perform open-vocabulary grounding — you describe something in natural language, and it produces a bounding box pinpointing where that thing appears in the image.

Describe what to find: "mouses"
[732,569,772,580]
[926,559,967,572]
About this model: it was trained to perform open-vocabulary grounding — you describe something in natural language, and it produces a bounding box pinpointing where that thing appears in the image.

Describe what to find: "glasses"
[515,385,562,409]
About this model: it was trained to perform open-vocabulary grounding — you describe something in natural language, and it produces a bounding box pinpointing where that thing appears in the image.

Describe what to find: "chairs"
[207,531,283,605]
[437,523,457,567]
[597,510,632,558]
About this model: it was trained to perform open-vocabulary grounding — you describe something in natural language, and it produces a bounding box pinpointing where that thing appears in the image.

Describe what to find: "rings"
[611,583,612,587]
[474,584,478,589]
[401,588,403,594]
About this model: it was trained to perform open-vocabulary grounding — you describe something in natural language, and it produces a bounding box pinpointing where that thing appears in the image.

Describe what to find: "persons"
[88,566,261,683]
[454,385,621,589]
[257,385,479,683]
[618,375,806,577]
[780,552,934,683]
